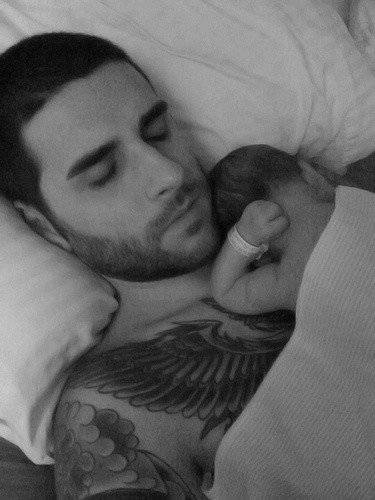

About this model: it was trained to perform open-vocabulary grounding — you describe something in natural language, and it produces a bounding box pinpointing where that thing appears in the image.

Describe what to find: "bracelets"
[227,223,270,260]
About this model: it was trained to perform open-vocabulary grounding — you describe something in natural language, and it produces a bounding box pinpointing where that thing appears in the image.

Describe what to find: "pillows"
[0,0,375,466]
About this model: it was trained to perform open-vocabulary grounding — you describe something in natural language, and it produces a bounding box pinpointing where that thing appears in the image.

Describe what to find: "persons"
[0,31,296,500]
[208,143,364,315]
